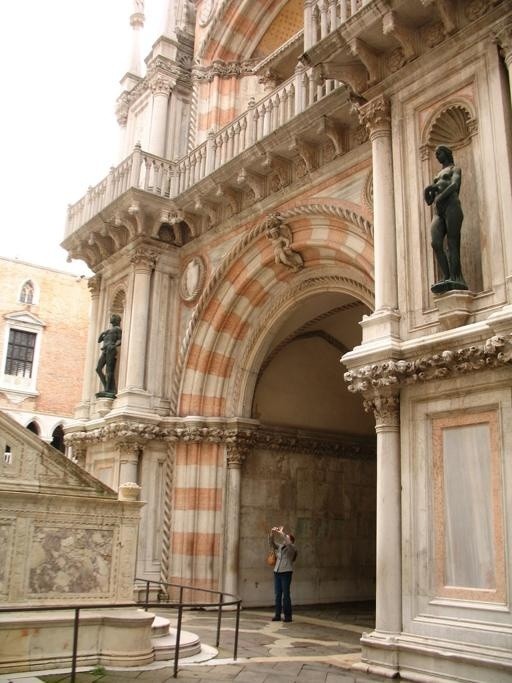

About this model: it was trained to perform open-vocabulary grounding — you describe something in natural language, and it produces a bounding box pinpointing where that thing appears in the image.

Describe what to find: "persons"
[263,228,299,275]
[93,313,122,401]
[423,142,471,295]
[265,525,299,624]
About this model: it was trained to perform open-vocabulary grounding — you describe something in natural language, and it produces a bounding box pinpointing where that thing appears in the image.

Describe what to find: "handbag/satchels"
[266,551,276,566]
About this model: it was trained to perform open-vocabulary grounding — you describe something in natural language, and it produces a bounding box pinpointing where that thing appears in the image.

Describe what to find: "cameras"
[272,527,279,532]
